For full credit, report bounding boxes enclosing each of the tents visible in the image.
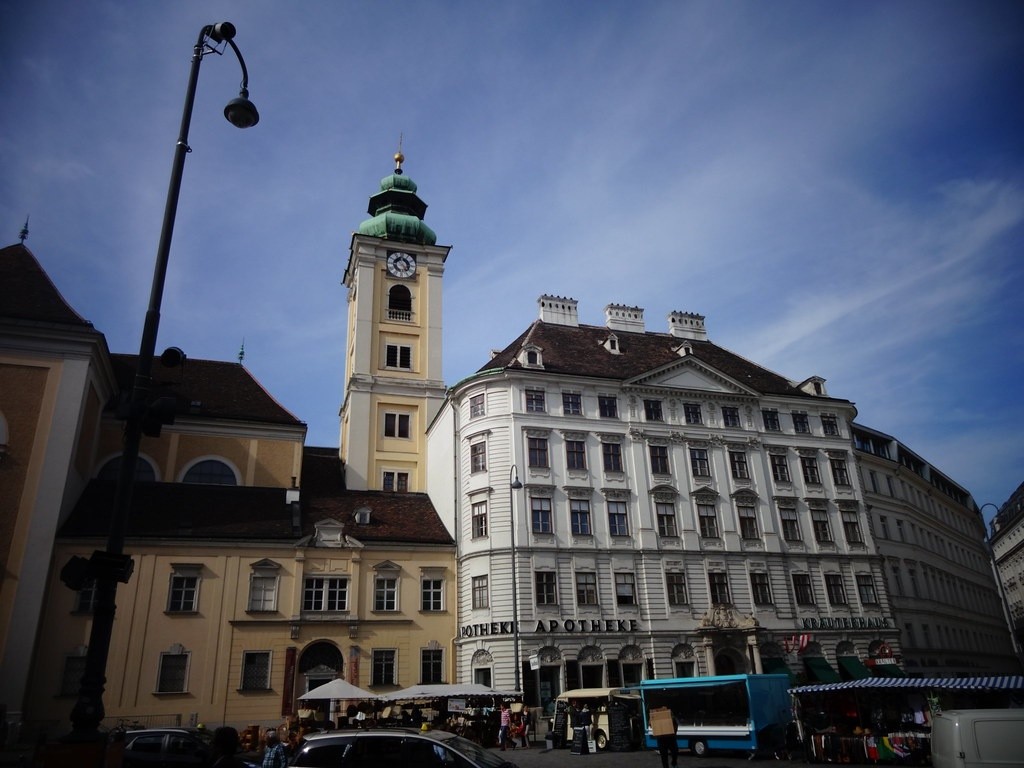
[377,683,524,701]
[788,676,1024,743]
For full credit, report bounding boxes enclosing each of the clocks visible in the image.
[386,252,416,278]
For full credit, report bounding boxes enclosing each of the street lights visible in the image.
[978,503,1024,665]
[509,464,523,702]
[66,19,260,768]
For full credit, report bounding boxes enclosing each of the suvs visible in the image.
[288,727,518,768]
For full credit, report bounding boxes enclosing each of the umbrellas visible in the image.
[297,679,376,722]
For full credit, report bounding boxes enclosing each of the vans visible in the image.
[548,688,641,748]
[930,707,1024,768]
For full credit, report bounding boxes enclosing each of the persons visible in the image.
[196,723,212,744]
[521,705,537,749]
[499,702,517,751]
[570,699,597,755]
[105,732,125,768]
[400,703,427,728]
[262,728,288,768]
[208,727,242,768]
[656,711,678,768]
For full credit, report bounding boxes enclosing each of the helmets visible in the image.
[197,723,205,728]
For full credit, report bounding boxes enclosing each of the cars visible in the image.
[117,730,257,768]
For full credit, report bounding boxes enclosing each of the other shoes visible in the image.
[512,742,517,750]
[499,747,505,751]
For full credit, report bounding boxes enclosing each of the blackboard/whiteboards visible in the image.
[570,730,585,754]
